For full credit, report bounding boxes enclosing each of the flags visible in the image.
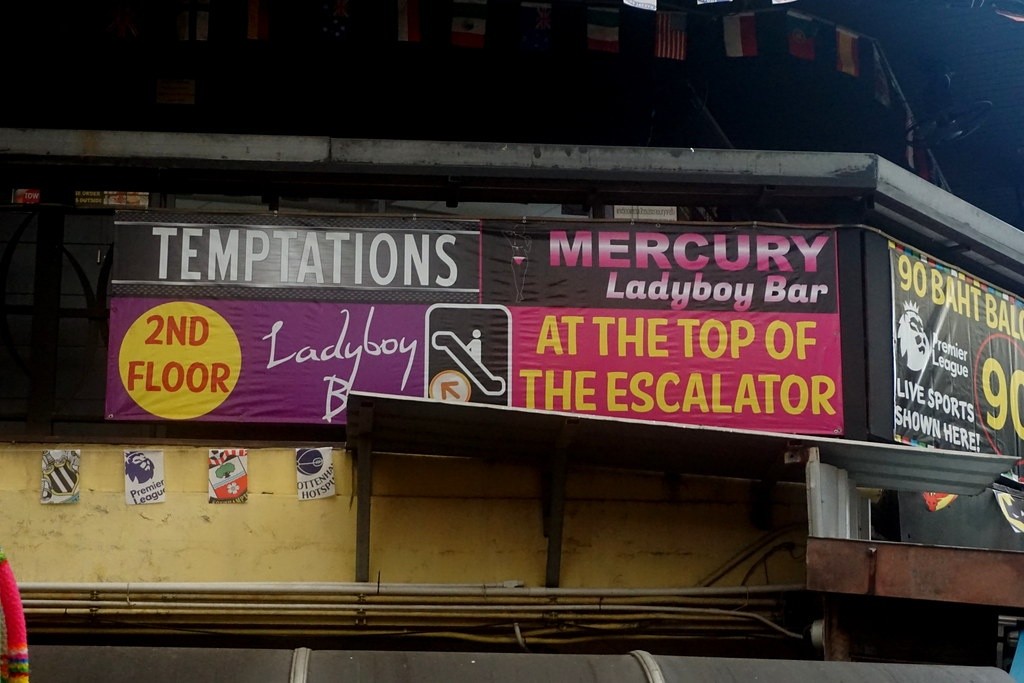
[722,12,758,58]
[872,45,931,181]
[449,0,490,50]
[321,0,353,39]
[785,11,816,60]
[834,27,861,77]
[656,10,688,60]
[519,0,554,53]
[111,0,278,43]
[586,6,621,53]
[397,0,428,45]
